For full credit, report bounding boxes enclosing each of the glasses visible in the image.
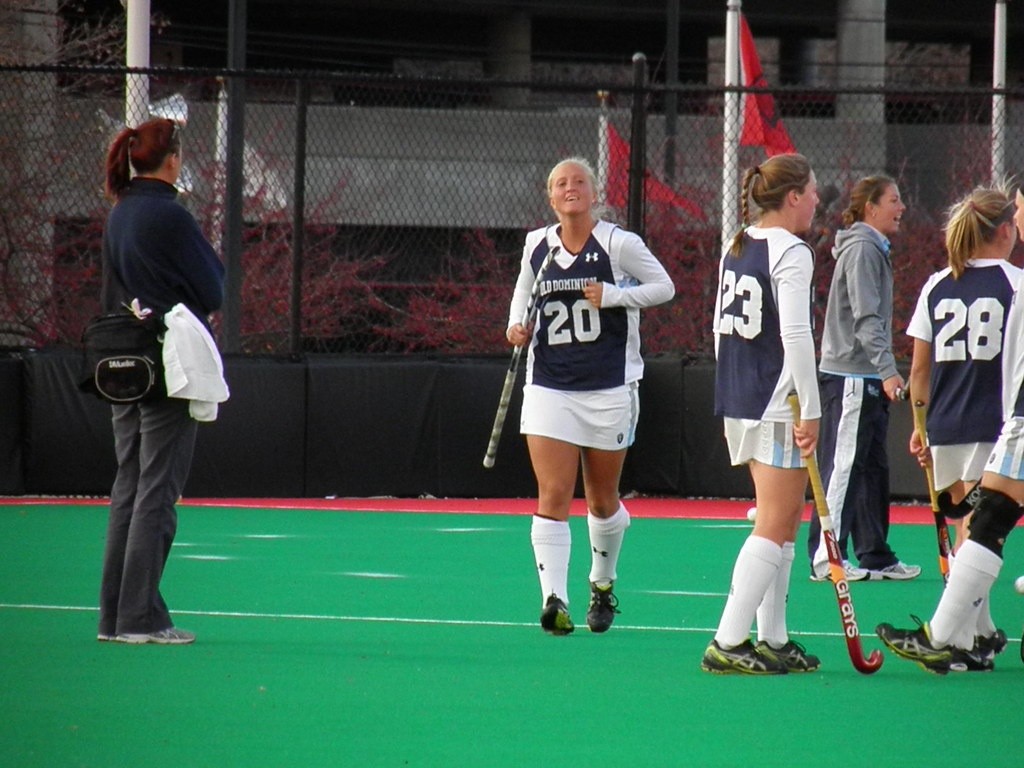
[167,118,180,144]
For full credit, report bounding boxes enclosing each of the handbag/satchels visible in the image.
[84,308,186,404]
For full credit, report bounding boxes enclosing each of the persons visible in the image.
[701,151,823,674]
[505,155,676,634]
[905,174,1024,672]
[874,178,1024,677]
[806,171,922,582]
[96,116,226,644]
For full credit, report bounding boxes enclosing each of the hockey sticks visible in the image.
[936,477,983,516]
[480,244,561,470]
[914,399,950,589]
[788,388,885,675]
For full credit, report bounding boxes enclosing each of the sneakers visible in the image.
[587,579,621,633]
[754,639,821,672]
[97,632,116,640]
[699,638,788,676]
[875,614,956,675]
[868,560,922,581]
[541,593,574,636]
[972,628,1007,657]
[810,560,871,582]
[949,644,993,672]
[117,626,195,644]
